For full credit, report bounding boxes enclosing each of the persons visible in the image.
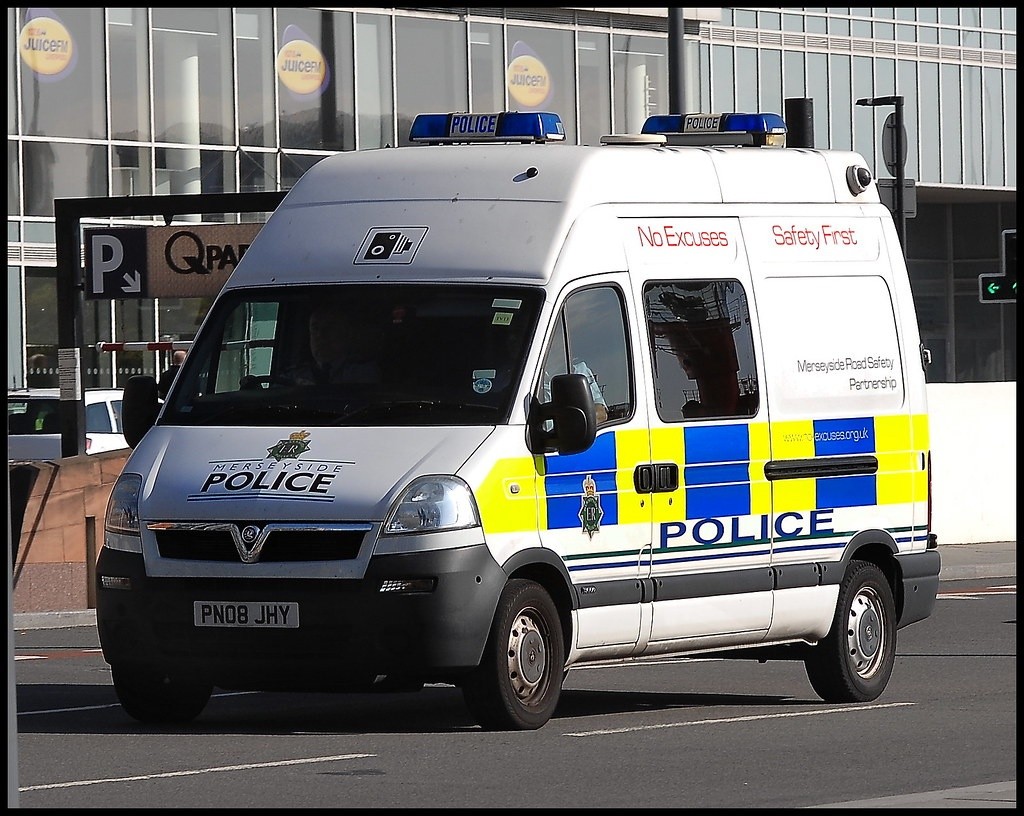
[543,334,610,427]
[158,350,187,412]
[239,300,381,390]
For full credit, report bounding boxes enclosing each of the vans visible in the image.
[93,110,941,732]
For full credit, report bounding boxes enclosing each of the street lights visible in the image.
[854,94,906,263]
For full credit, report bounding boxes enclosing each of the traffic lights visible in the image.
[978,231,1016,302]
